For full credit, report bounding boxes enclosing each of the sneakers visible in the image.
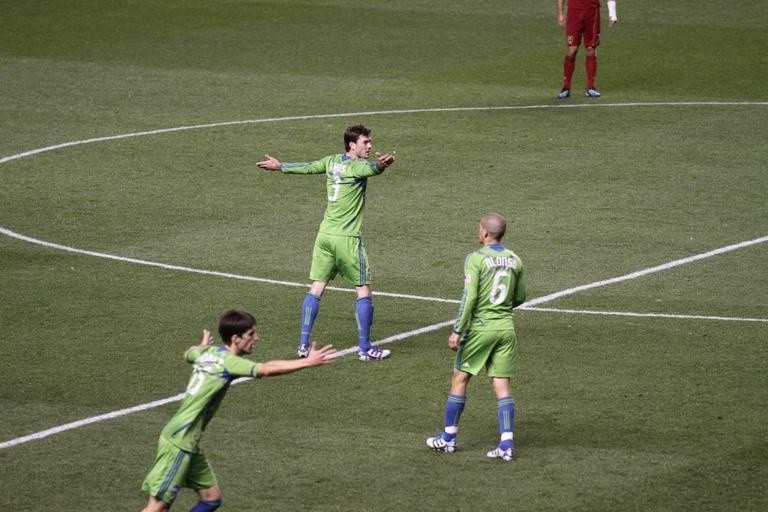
[426,436,458,454]
[356,344,392,361]
[486,446,516,461]
[585,88,601,97]
[296,343,323,358]
[557,88,570,99]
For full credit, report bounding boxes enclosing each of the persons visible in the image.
[139,306,337,512]
[253,123,396,363]
[553,0,618,100]
[424,211,526,461]
